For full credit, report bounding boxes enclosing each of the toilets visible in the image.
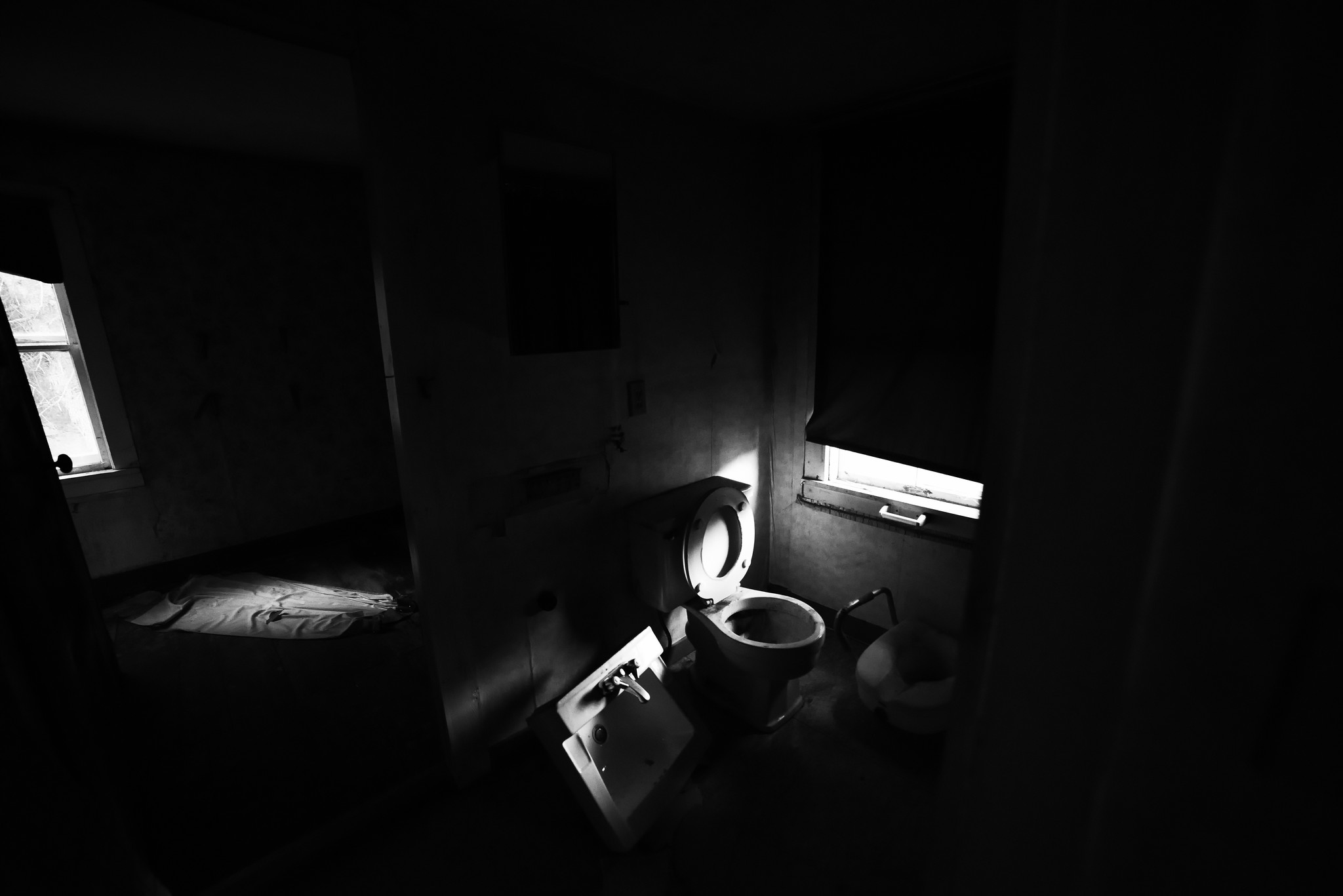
[625,476,826,736]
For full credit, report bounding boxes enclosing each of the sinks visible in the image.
[533,624,698,852]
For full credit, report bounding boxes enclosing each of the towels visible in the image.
[123,570,399,641]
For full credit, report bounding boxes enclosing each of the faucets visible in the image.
[599,659,652,705]
